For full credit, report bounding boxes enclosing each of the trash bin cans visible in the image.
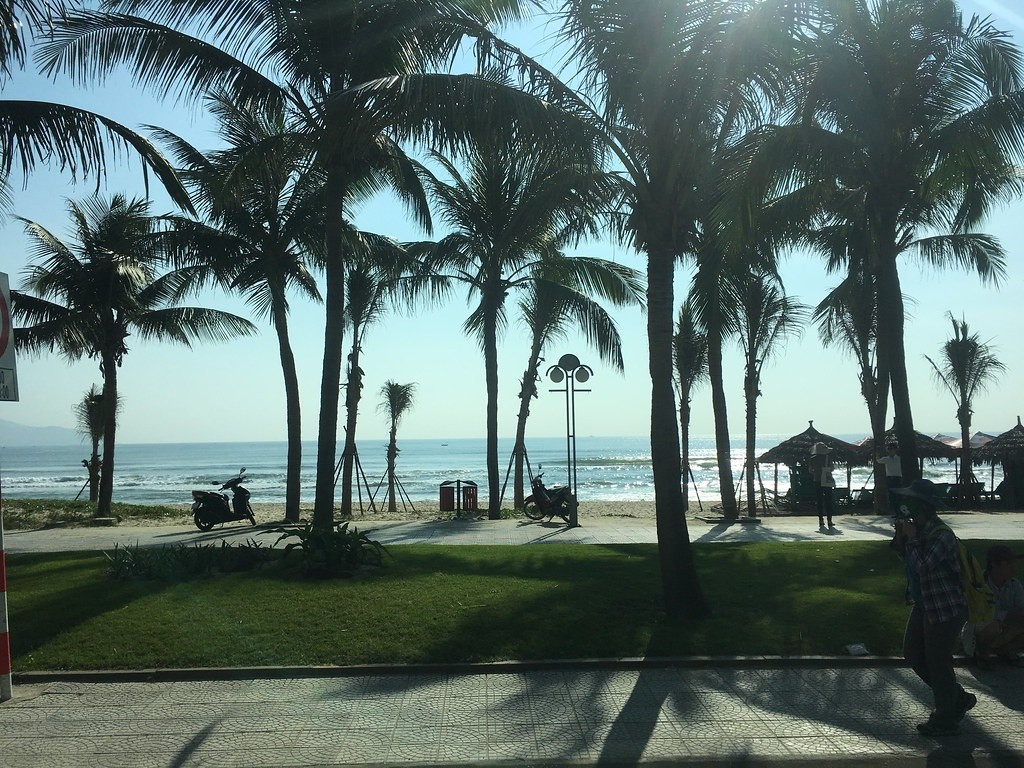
[440,480,478,511]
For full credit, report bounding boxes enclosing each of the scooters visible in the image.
[189,467,256,532]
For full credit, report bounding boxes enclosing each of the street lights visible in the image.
[544,354,594,528]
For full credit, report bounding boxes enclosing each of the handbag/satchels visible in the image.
[821,455,835,487]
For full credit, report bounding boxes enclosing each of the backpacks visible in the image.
[926,525,996,625]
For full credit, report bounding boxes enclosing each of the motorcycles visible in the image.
[522,462,579,523]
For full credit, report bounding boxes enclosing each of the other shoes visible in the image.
[1003,647,1024,667]
[828,522,835,526]
[917,719,960,736]
[819,522,825,525]
[929,684,976,722]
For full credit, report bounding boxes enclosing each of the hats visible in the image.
[889,479,935,504]
[985,544,1024,561]
[811,442,833,455]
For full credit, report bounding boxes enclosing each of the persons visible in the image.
[807,441,836,526]
[875,443,903,518]
[961,543,1024,668]
[889,478,977,735]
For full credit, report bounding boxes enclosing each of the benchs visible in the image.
[933,481,985,505]
[836,488,850,504]
